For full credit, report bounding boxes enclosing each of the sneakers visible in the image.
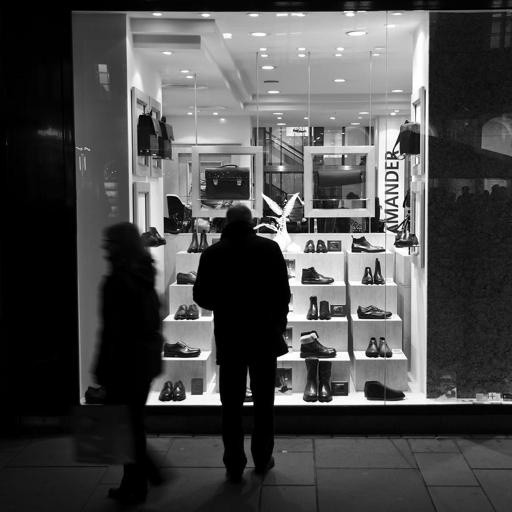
[225,455,274,483]
[108,486,147,503]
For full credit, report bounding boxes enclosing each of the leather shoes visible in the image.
[366,337,392,358]
[351,236,385,252]
[304,240,326,253]
[177,273,197,285]
[159,381,185,401]
[141,227,166,247]
[357,305,391,319]
[174,304,199,320]
[364,380,404,399]
[163,340,200,357]
[302,267,333,285]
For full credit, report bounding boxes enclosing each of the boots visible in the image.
[306,296,331,320]
[300,331,336,402]
[188,229,208,253]
[363,258,385,284]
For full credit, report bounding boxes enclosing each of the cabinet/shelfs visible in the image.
[144,233,408,406]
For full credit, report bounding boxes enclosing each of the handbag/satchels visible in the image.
[394,216,418,248]
[138,109,174,160]
[264,183,287,216]
[205,164,250,199]
[317,170,362,199]
[398,120,420,155]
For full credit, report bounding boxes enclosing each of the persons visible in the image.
[191,205,293,483]
[89,220,178,505]
[433,183,512,213]
